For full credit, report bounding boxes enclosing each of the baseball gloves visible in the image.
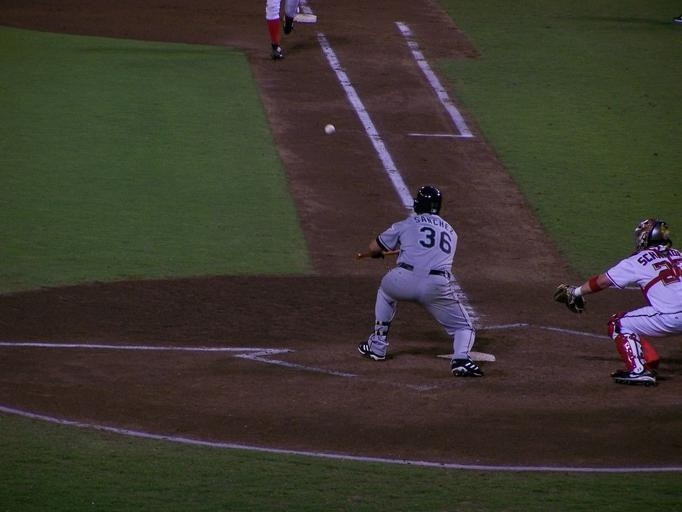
[553,283,587,313]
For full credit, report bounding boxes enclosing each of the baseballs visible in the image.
[325,124,335,134]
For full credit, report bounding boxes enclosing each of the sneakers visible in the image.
[450,358,484,377]
[271,43,283,58]
[357,341,387,361]
[283,20,294,34]
[610,369,657,386]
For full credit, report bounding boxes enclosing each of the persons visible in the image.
[566,218,682,386]
[265,0,300,60]
[357,185,484,377]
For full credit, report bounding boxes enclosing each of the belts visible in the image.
[399,262,446,277]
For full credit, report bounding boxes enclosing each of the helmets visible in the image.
[413,186,441,213]
[634,220,669,249]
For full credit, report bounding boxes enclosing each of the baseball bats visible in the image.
[356,249,400,258]
